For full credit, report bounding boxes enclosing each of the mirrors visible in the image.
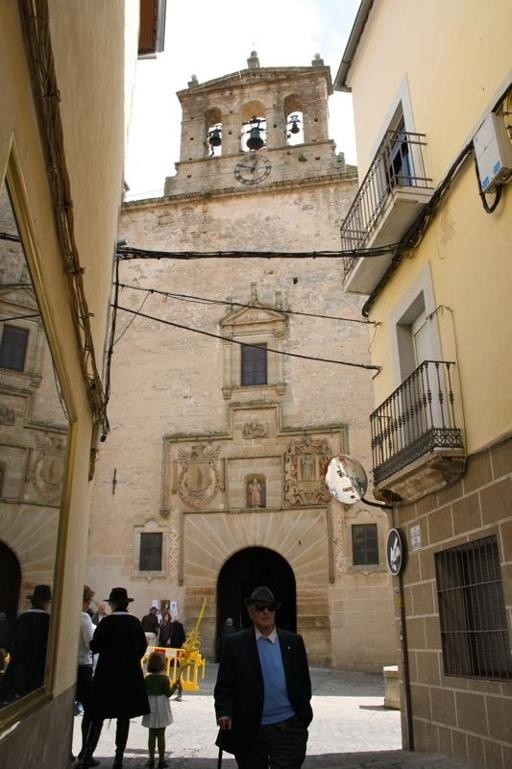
[324,455,368,505]
[0,135,81,734]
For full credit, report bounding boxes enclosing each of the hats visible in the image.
[103,587,135,603]
[26,584,53,600]
[244,587,278,606]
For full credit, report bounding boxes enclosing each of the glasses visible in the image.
[255,602,275,613]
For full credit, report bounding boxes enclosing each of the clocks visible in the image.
[233,153,271,185]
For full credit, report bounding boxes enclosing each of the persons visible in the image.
[0,583,190,768]
[301,452,316,482]
[248,476,265,509]
[210,584,316,769]
